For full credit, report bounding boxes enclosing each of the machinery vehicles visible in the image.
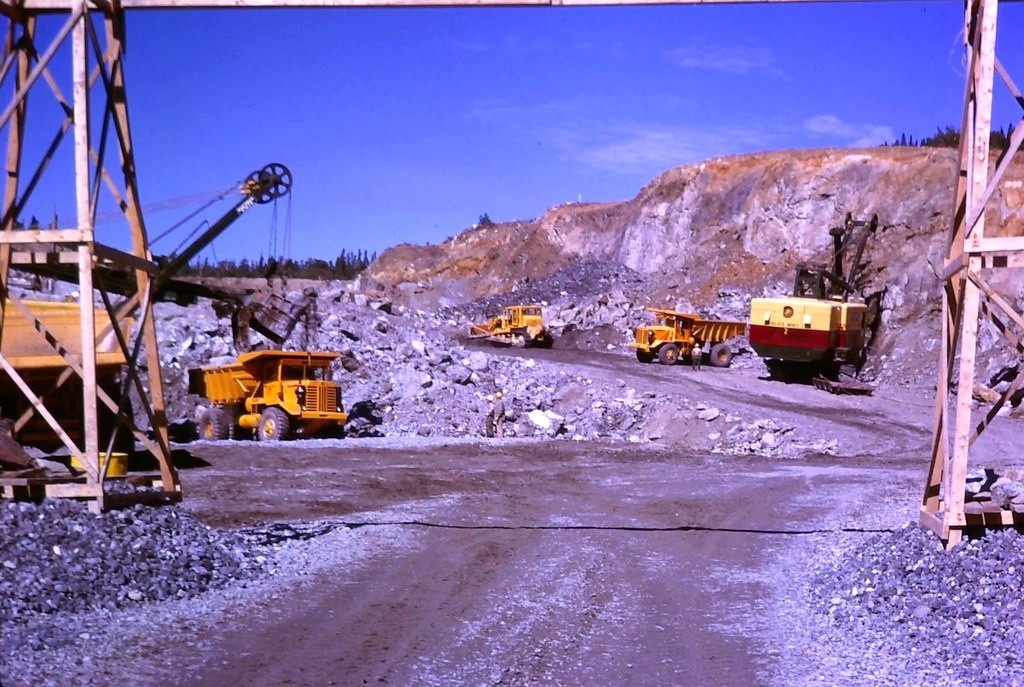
[466,305,547,349]
[628,306,748,368]
[748,211,886,385]
[184,346,349,445]
